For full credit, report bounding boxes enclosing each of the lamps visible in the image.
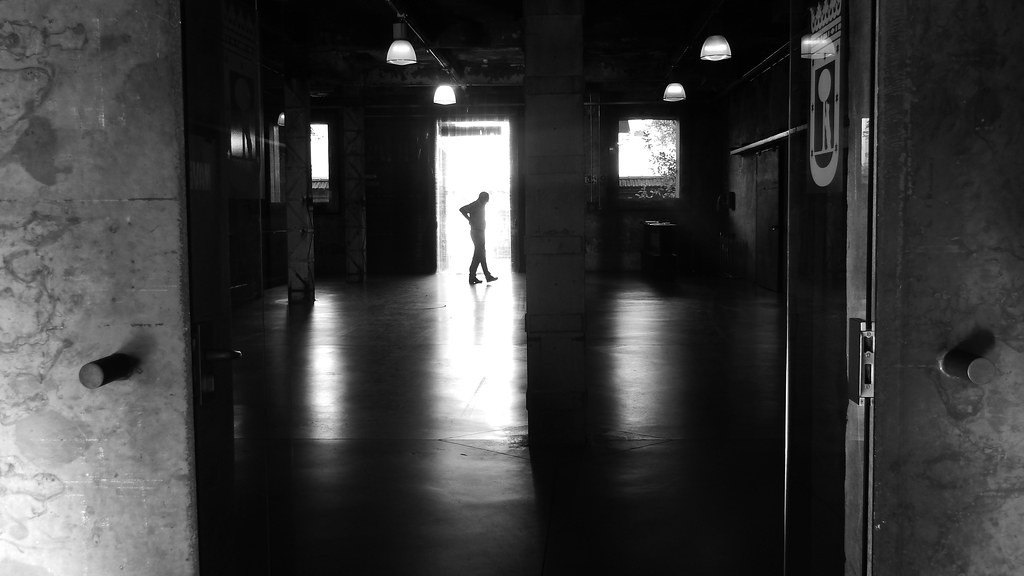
[433,67,457,105]
[700,8,732,61]
[386,13,417,65]
[661,63,686,103]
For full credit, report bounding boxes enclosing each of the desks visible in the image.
[641,224,677,273]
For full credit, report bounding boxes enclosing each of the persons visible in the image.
[459,192,498,285]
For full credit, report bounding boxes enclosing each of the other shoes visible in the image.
[469,277,482,283]
[486,275,498,282]
